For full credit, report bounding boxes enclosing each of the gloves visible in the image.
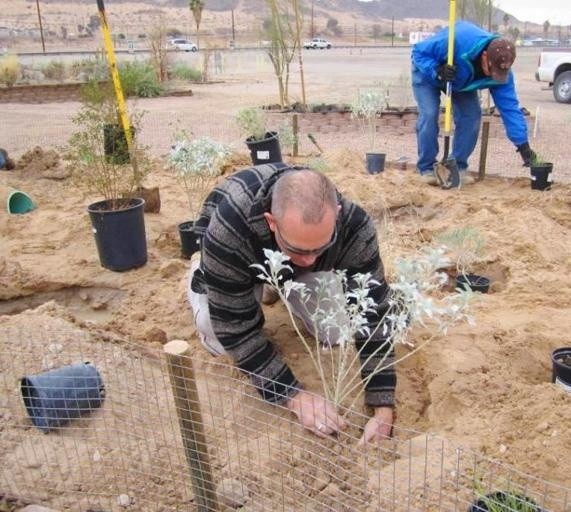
[436,64,457,82]
[516,142,537,167]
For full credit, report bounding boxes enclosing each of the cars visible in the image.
[166,39,197,52]
[536,51,571,103]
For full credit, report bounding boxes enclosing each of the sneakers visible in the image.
[423,169,475,186]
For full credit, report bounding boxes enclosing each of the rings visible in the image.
[318,424,324,430]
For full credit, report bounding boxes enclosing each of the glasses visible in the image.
[277,225,338,256]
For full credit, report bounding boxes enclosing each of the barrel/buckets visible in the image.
[0,148,15,171]
[455,274,492,294]
[366,152,386,174]
[245,133,281,166]
[89,197,147,270]
[527,162,554,191]
[178,221,199,257]
[103,123,135,166]
[7,191,34,215]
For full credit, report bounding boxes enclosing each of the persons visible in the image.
[411,20,537,187]
[182,161,398,446]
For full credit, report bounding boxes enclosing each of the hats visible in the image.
[486,37,516,82]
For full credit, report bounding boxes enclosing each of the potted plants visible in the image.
[469,480,543,511]
[68,63,151,273]
[435,226,491,293]
[351,88,392,175]
[528,151,554,192]
[161,133,230,258]
[233,102,283,166]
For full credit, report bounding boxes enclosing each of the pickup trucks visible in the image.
[304,38,331,50]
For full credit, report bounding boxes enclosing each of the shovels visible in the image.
[433,0,460,190]
[96,0,161,214]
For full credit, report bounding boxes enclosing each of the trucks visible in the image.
[410,32,434,45]
